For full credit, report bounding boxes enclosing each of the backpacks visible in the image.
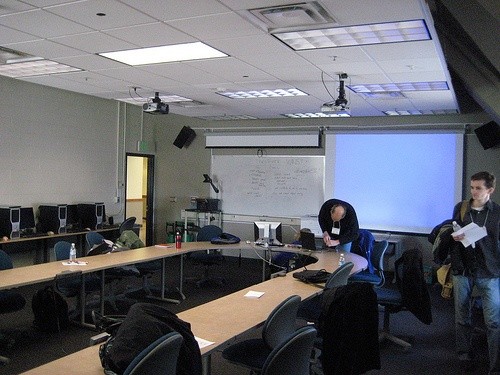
[32,285,69,330]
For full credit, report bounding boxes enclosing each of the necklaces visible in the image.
[470,209,489,226]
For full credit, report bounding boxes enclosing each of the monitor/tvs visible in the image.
[254,222,283,248]
[67,205,78,224]
[20,208,35,229]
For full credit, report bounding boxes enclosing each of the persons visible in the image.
[440,172,500,375]
[318,199,359,252]
[265,228,311,281]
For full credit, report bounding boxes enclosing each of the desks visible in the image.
[0,224,142,265]
[0,242,368,375]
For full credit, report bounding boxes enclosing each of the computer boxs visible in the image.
[39,205,67,234]
[78,204,103,230]
[0,208,20,240]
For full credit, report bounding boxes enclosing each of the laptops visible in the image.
[300,232,326,251]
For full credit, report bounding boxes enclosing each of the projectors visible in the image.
[143,102,169,115]
[321,105,351,115]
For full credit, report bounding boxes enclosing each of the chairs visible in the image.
[0,217,423,375]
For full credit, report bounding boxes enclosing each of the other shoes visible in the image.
[458,351,475,370]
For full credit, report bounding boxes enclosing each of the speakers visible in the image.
[173,126,197,150]
[473,121,500,150]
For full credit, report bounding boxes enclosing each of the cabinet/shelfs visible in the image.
[184,208,221,242]
[166,222,194,243]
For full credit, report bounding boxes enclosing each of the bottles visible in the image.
[338,253,346,267]
[452,221,461,232]
[70,243,76,263]
[175,232,181,249]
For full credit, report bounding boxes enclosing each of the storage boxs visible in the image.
[196,198,220,210]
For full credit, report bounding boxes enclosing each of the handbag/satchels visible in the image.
[293,269,332,284]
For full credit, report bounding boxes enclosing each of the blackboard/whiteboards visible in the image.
[210,155,326,217]
